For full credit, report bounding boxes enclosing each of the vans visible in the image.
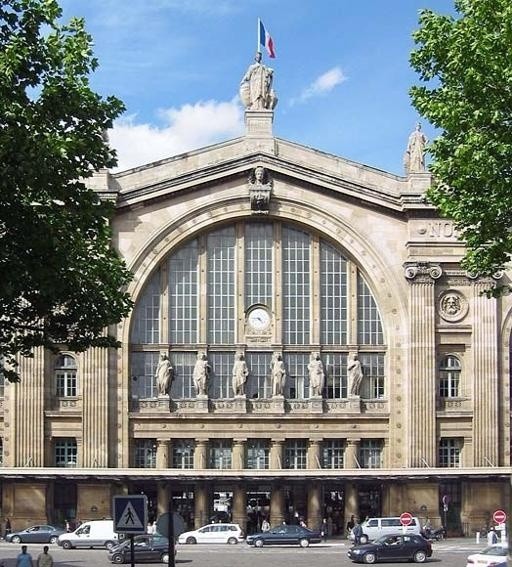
[349,517,422,544]
[57,521,122,550]
[178,523,245,545]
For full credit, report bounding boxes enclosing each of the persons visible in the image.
[305,352,325,396]
[485,525,499,546]
[347,354,364,396]
[77,520,83,533]
[153,351,173,396]
[442,295,460,314]
[65,519,74,532]
[270,354,286,396]
[252,165,265,185]
[146,512,434,546]
[1,517,12,539]
[36,545,54,566]
[193,351,209,399]
[243,52,278,109]
[405,122,430,171]
[16,544,35,566]
[230,351,249,396]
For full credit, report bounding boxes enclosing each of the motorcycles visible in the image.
[420,516,446,542]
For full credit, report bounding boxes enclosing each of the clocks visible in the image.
[244,302,273,335]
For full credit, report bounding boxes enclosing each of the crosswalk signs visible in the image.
[114,496,146,534]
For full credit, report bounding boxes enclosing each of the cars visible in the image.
[246,525,323,549]
[466,543,510,567]
[4,525,72,545]
[347,534,433,564]
[108,535,177,564]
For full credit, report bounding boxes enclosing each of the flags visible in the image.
[260,20,275,59]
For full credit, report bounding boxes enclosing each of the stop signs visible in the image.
[401,513,413,525]
[494,510,506,524]
